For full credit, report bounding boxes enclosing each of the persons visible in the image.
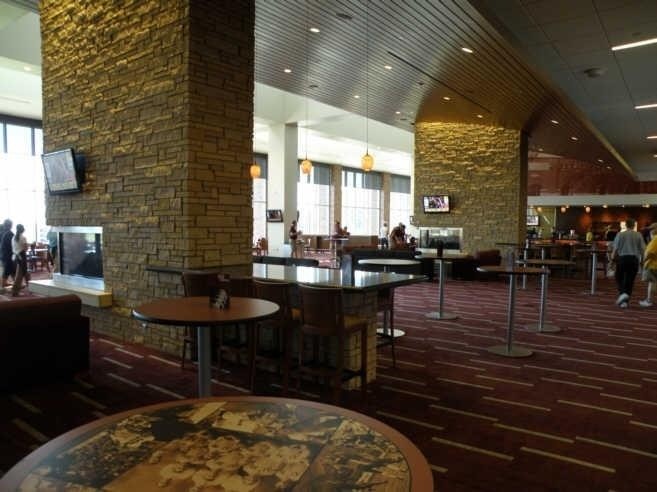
[334,221,343,238]
[608,217,646,308]
[405,237,416,260]
[0,219,26,293]
[9,223,27,297]
[635,228,656,309]
[341,226,350,238]
[389,224,406,249]
[604,223,617,265]
[45,229,57,266]
[287,220,301,259]
[640,219,651,249]
[379,223,388,250]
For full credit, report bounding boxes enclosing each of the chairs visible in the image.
[182,273,368,414]
[376,271,396,368]
[0,294,90,396]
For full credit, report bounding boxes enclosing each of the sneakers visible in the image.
[616,293,654,308]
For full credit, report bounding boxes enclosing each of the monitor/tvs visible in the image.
[41,149,81,195]
[423,194,451,214]
[526,215,540,226]
[266,209,283,222]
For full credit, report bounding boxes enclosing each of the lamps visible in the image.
[362,0,373,172]
[300,0,312,175]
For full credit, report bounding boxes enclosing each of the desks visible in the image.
[323,238,349,261]
[415,254,468,320]
[0,395,435,492]
[358,259,421,338]
[476,243,612,358]
[131,296,280,398]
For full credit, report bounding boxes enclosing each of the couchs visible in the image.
[351,248,502,281]
